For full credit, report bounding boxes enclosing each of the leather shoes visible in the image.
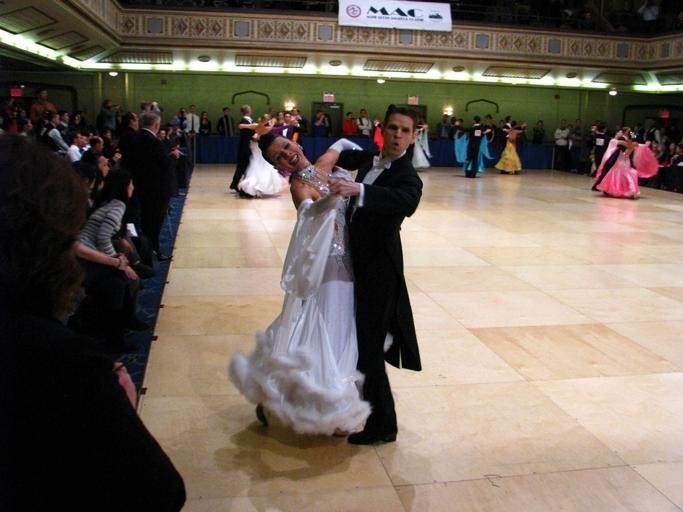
[348,427,398,446]
[230,184,239,192]
[158,253,172,262]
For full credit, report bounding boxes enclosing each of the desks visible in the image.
[197,133,557,169]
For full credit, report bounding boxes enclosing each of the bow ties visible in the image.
[373,155,392,171]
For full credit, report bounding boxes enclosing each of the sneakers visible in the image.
[110,339,140,354]
[133,263,155,280]
[126,316,150,333]
